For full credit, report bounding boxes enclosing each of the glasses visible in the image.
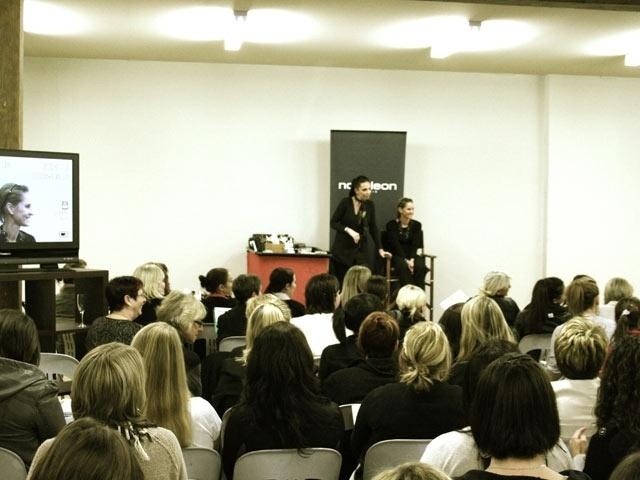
[194,320,204,327]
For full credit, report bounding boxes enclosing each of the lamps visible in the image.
[429,21,483,59]
[223,9,250,52]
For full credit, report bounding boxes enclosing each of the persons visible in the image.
[0,182,38,245]
[386,198,428,289]
[330,175,393,277]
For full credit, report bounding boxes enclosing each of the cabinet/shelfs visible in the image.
[0,264,110,362]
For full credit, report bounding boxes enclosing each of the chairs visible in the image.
[378,231,436,322]
[2,300,639,480]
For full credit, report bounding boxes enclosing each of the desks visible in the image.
[248,247,333,302]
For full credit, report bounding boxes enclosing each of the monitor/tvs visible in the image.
[0,148,81,273]
